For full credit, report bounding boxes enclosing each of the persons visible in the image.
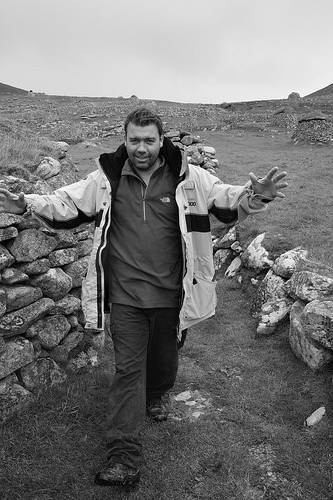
[0,108,288,487]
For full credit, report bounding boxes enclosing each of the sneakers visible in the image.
[96,463,140,486]
[148,394,168,421]
